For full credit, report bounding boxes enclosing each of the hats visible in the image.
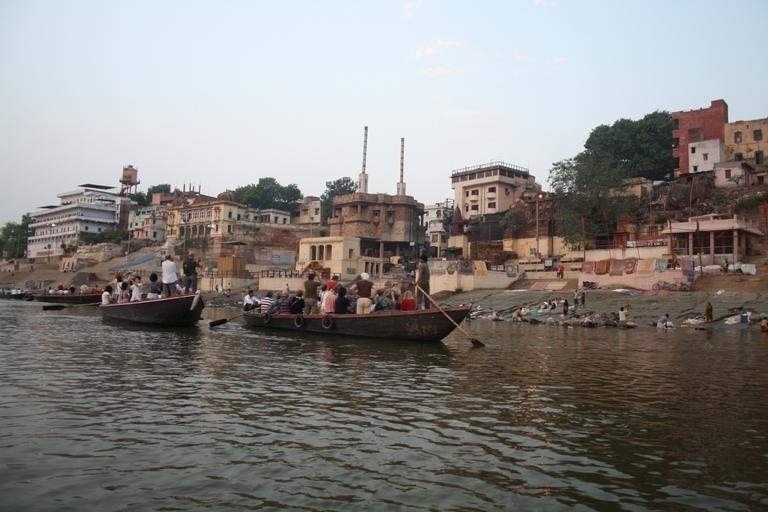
[361,272,369,280]
[418,255,427,261]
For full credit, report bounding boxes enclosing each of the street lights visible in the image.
[535,193,543,270]
[45,222,57,265]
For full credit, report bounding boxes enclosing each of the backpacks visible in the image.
[375,298,384,311]
[183,261,195,275]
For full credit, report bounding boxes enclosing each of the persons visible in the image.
[705,301,714,322]
[706,323,713,342]
[242,290,260,310]
[161,253,178,296]
[488,288,629,329]
[6,283,103,296]
[101,270,192,306]
[761,317,768,333]
[559,261,565,278]
[269,272,417,316]
[182,253,204,293]
[412,254,431,310]
[0,287,5,297]
[671,252,678,269]
[258,292,275,314]
[656,314,669,328]
